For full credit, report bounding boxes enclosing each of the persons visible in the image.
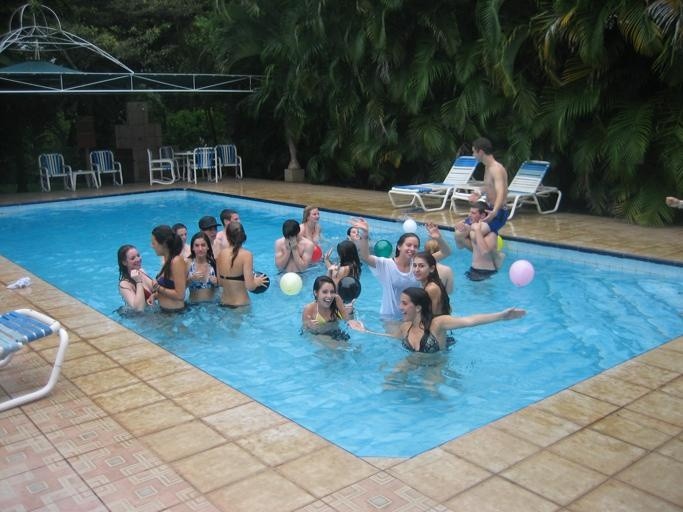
[116,209,269,314]
[324,240,362,291]
[347,287,527,391]
[666,196,683,210]
[345,216,451,317]
[299,206,322,245]
[301,276,355,348]
[347,227,361,241]
[454,201,498,280]
[454,136,509,268]
[275,220,314,273]
[411,251,452,316]
[424,239,454,295]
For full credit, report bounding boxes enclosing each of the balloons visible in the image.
[374,239,393,258]
[403,219,418,233]
[509,259,536,287]
[496,235,504,252]
[279,272,303,296]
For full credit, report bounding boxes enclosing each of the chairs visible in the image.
[39,153,74,192]
[388,156,562,220]
[0,309,68,411]
[147,144,243,186]
[89,151,123,187]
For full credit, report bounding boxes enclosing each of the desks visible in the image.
[72,171,99,192]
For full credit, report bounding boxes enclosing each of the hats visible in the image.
[198,215,222,230]
